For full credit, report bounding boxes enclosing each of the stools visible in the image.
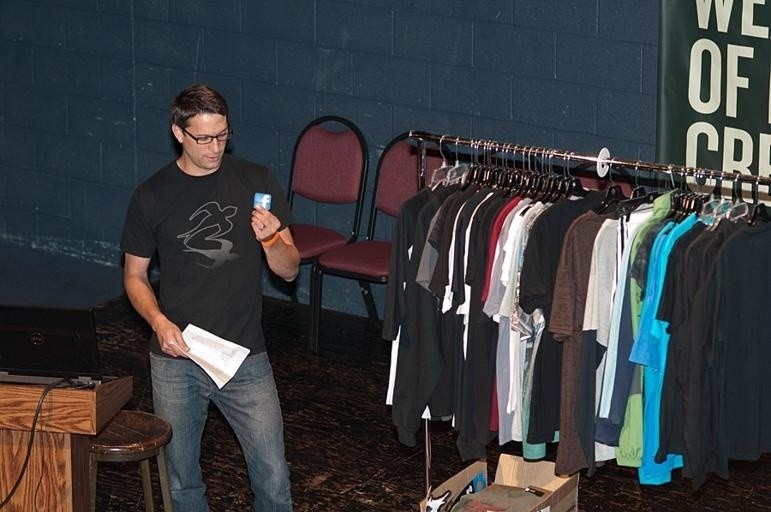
[87,410,173,512]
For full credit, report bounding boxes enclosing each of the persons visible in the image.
[121,83,301,512]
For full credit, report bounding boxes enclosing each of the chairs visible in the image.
[288,115,374,350]
[312,130,447,354]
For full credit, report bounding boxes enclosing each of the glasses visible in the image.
[179,126,234,146]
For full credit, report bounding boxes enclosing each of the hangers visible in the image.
[428,134,771,231]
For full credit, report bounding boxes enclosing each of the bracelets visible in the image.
[261,231,280,247]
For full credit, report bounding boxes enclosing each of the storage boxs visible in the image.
[419,453,580,512]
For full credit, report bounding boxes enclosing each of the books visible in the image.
[167,321,253,391]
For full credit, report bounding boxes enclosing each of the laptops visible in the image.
[0,303,118,387]
